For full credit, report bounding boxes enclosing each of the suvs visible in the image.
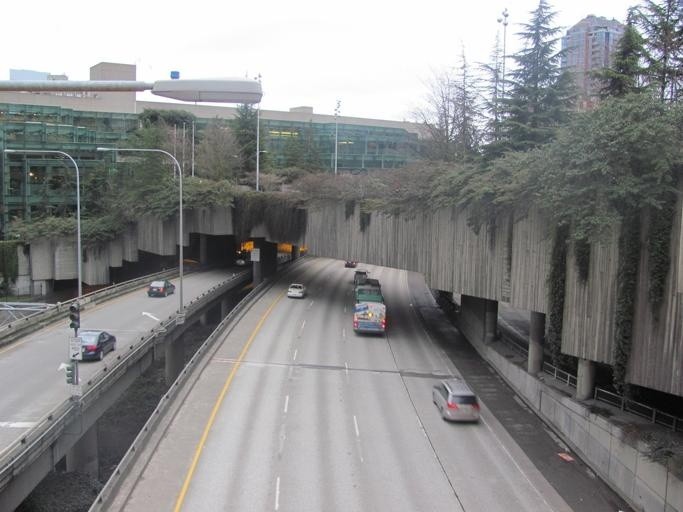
[432,378,481,426]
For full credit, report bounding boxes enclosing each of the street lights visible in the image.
[0,77,264,110]
[251,74,266,192]
[332,97,346,177]
[2,147,85,298]
[95,145,185,314]
[497,8,508,142]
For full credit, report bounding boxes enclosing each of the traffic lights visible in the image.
[69,302,78,330]
[65,365,72,383]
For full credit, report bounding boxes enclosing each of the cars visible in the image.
[344,260,358,268]
[76,329,116,363]
[145,280,175,297]
[287,283,306,298]
[352,268,386,335]
[231,249,251,267]
